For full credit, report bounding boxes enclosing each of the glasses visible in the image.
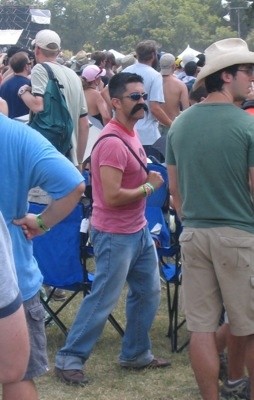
[233,68,254,75]
[119,93,148,100]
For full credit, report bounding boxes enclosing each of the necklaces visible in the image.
[88,86,95,89]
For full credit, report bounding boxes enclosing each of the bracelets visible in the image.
[35,214,50,232]
[141,183,154,197]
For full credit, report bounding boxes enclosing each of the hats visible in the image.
[31,29,61,51]
[160,53,176,75]
[192,38,254,91]
[82,65,106,83]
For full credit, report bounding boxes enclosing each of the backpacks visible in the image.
[29,63,74,155]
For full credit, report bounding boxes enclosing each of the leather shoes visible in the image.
[55,366,85,386]
[147,358,171,368]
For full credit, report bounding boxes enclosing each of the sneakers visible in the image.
[222,376,249,395]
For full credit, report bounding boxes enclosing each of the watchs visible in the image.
[18,88,29,98]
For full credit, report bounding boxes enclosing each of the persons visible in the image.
[153,53,190,159]
[188,86,208,107]
[79,65,111,172]
[164,37,254,400]
[17,29,90,301]
[0,112,86,400]
[0,51,32,123]
[53,72,171,385]
[0,210,31,385]
[0,74,9,117]
[215,301,249,400]
[0,49,254,116]
[120,39,173,164]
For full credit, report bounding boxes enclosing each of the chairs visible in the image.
[26,164,191,352]
